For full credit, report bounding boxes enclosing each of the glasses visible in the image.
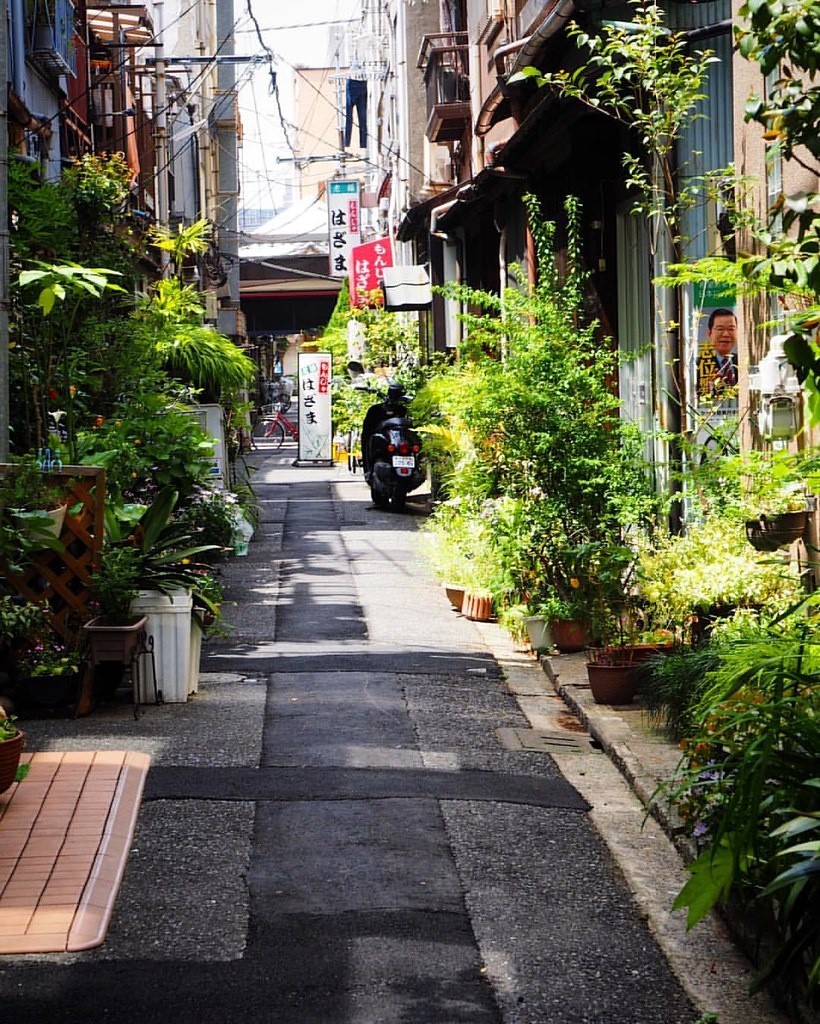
[712,325,736,334]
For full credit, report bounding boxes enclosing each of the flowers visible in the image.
[0,703,19,742]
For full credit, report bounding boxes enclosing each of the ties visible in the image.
[722,358,735,387]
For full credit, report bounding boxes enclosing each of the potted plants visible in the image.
[407,186,820,1023]
[81,547,151,719]
[1,147,263,704]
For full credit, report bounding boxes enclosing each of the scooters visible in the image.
[353,384,428,512]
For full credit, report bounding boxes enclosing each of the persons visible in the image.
[696,436,729,522]
[697,310,738,401]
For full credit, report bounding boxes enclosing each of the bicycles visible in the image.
[250,398,298,449]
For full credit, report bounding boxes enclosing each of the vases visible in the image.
[0,729,23,795]
